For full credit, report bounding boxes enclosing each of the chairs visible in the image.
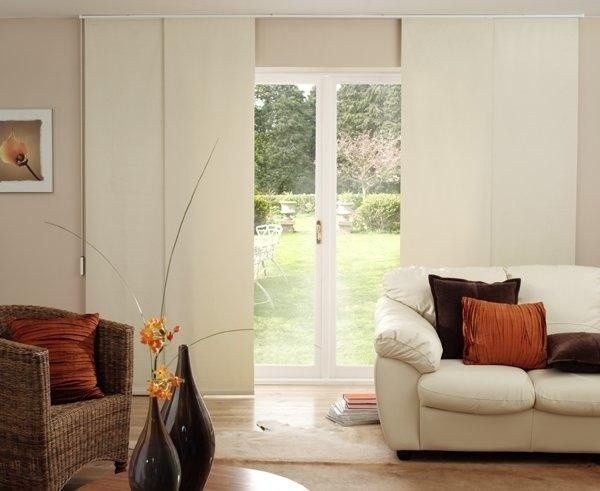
[0,301,137,490]
[254,223,289,310]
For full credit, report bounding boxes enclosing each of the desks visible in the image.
[75,461,312,490]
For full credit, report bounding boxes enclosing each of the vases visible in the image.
[160,343,218,491]
[124,393,187,491]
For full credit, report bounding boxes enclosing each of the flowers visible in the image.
[141,313,192,402]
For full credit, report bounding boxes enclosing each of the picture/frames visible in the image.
[0,106,57,192]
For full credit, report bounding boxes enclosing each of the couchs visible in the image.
[373,265,600,465]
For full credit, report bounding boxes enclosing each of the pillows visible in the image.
[6,312,105,403]
[428,273,522,363]
[545,331,600,375]
[458,294,549,368]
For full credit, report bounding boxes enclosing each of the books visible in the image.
[324,393,380,427]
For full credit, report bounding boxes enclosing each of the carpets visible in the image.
[129,419,598,490]
